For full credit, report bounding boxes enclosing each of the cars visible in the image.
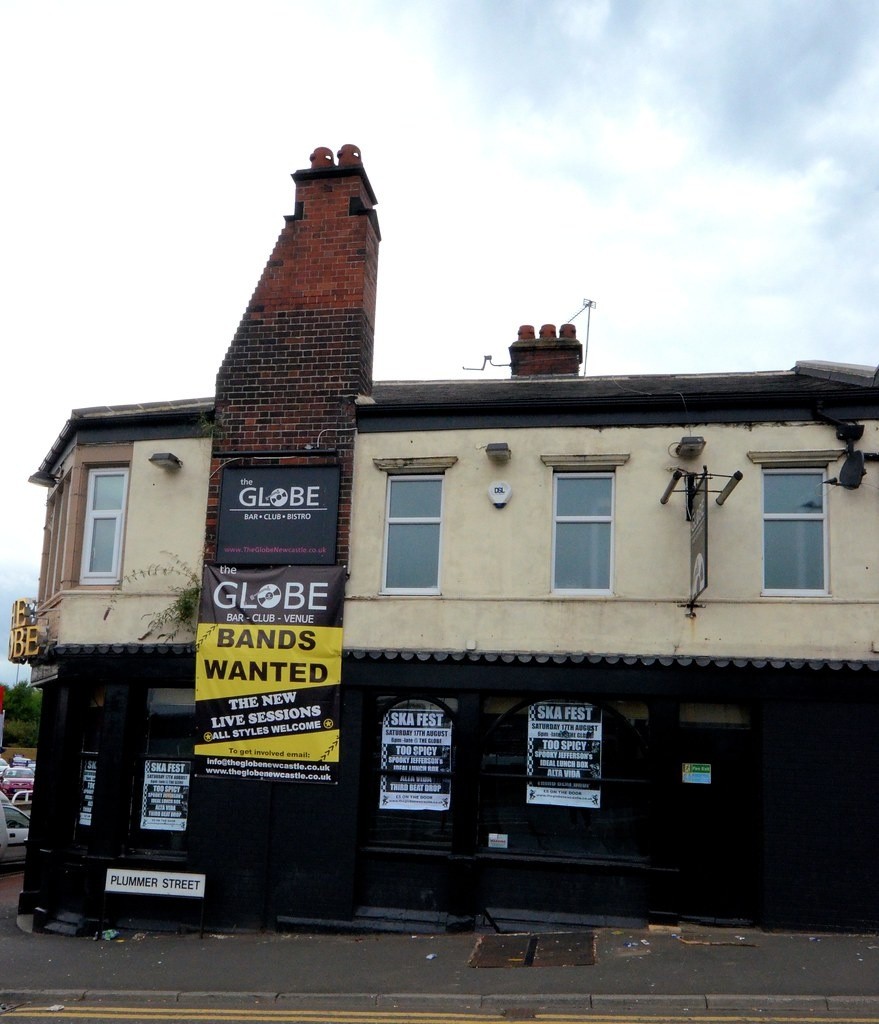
[0,806,30,865]
[0,758,10,779]
[10,755,37,773]
[0,767,35,800]
